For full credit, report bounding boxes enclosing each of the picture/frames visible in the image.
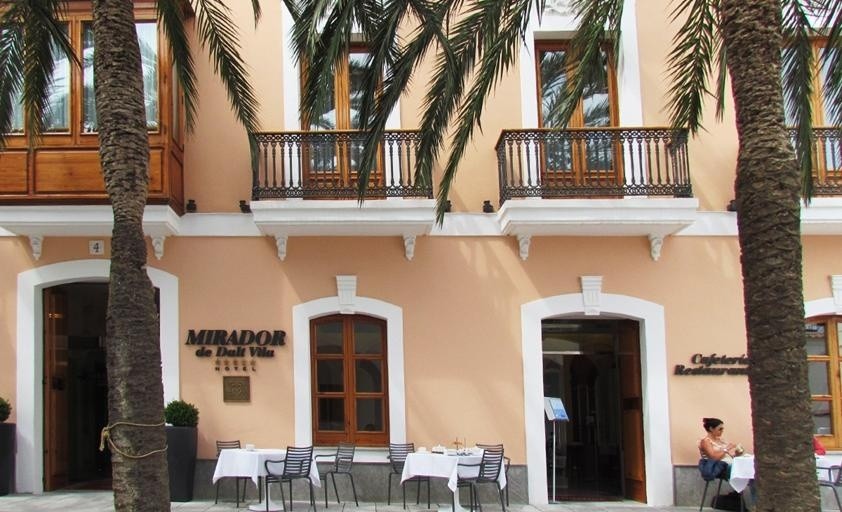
[223,376,250,401]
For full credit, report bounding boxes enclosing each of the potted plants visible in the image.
[164,400,199,502]
[0,398,16,495]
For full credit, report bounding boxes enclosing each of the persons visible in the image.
[813,437,825,456]
[698,417,736,480]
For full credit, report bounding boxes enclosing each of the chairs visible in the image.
[265,446,316,511]
[698,446,744,512]
[387,443,430,509]
[451,448,505,512]
[314,445,358,508]
[816,465,841,512]
[473,444,510,510]
[215,441,261,508]
